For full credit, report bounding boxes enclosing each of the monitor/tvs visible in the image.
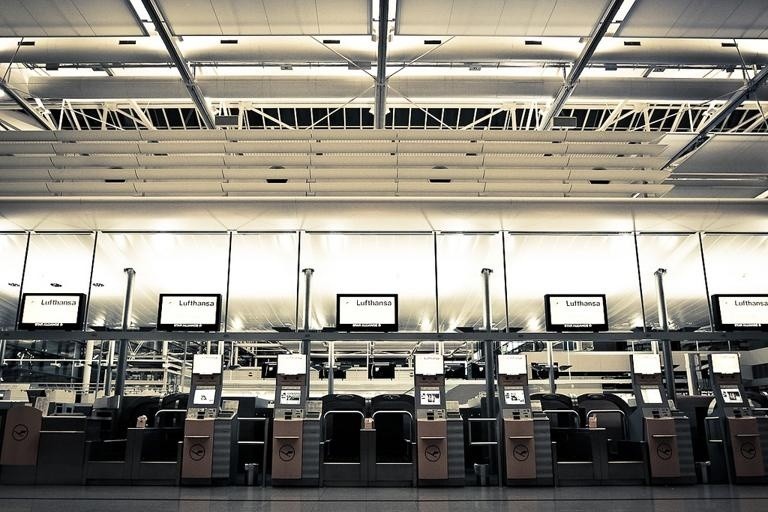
[262,364,277,378]
[319,365,346,378]
[720,388,744,404]
[640,388,663,404]
[444,362,465,378]
[701,360,710,379]
[472,362,486,378]
[95,394,121,417]
[27,389,46,407]
[504,390,526,404]
[420,390,441,406]
[532,362,558,379]
[193,389,216,405]
[280,390,301,405]
[368,362,394,378]
